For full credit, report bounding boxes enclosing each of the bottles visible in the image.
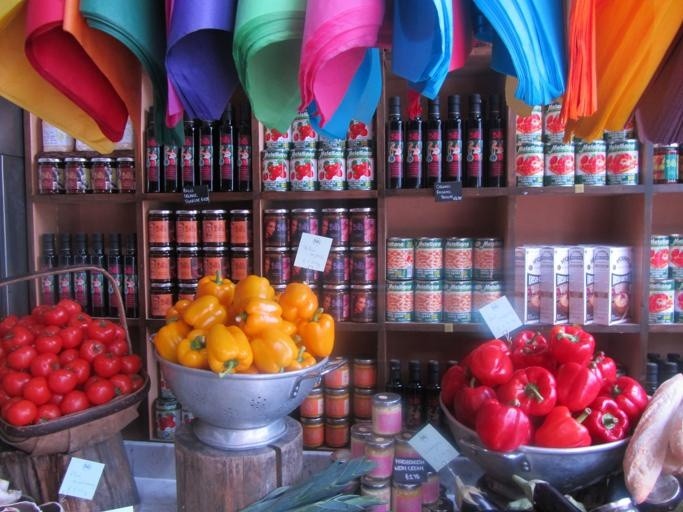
[386,358,460,428]
[145,102,254,194]
[38,231,139,320]
[385,89,509,189]
[643,351,682,397]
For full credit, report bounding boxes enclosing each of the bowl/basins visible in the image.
[142,334,346,449]
[439,394,634,502]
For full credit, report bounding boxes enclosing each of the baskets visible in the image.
[0,263,151,459]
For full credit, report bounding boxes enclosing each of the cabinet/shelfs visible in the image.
[22,49,683,453]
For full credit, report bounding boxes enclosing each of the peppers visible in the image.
[155,268,336,373]
[440,327,647,454]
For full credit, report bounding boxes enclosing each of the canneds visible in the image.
[641,475,683,511]
[653,142,680,184]
[516,103,640,187]
[152,366,195,441]
[37,156,138,194]
[261,110,375,191]
[148,209,254,317]
[263,207,376,324]
[302,358,440,511]
[386,237,502,325]
[648,233,683,325]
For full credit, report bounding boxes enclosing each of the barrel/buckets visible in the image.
[0,430,142,512]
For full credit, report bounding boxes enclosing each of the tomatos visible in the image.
[1,299,143,424]
